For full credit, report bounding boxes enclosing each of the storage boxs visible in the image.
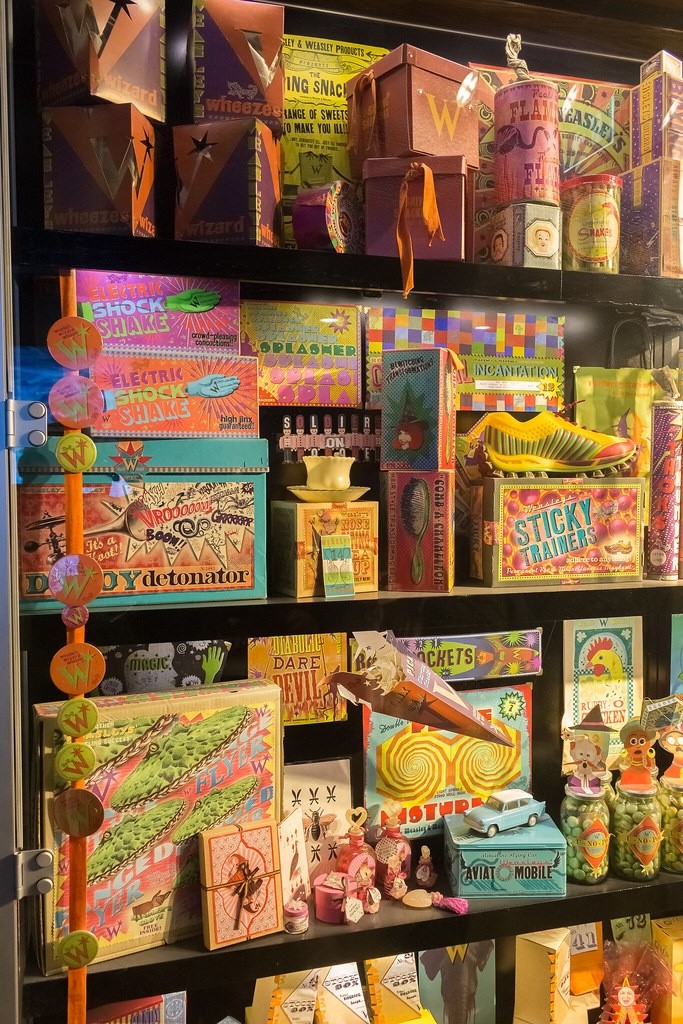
[78,911,683,1024]
[16,266,683,611]
[20,1,682,280]
[34,613,683,979]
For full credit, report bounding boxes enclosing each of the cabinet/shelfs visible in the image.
[0,0,683,1024]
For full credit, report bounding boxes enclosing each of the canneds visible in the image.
[559,769,683,886]
[493,79,622,275]
